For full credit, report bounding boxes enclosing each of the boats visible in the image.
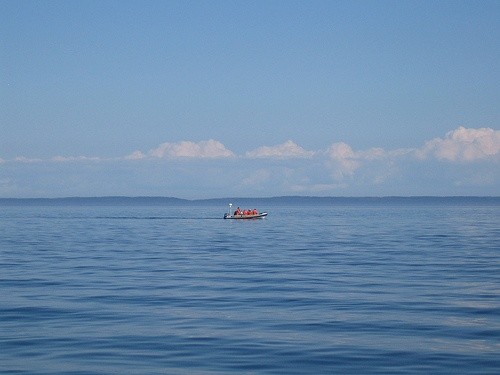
[225,203,268,219]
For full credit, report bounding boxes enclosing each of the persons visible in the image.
[253,208,259,214]
[235,206,254,215]
